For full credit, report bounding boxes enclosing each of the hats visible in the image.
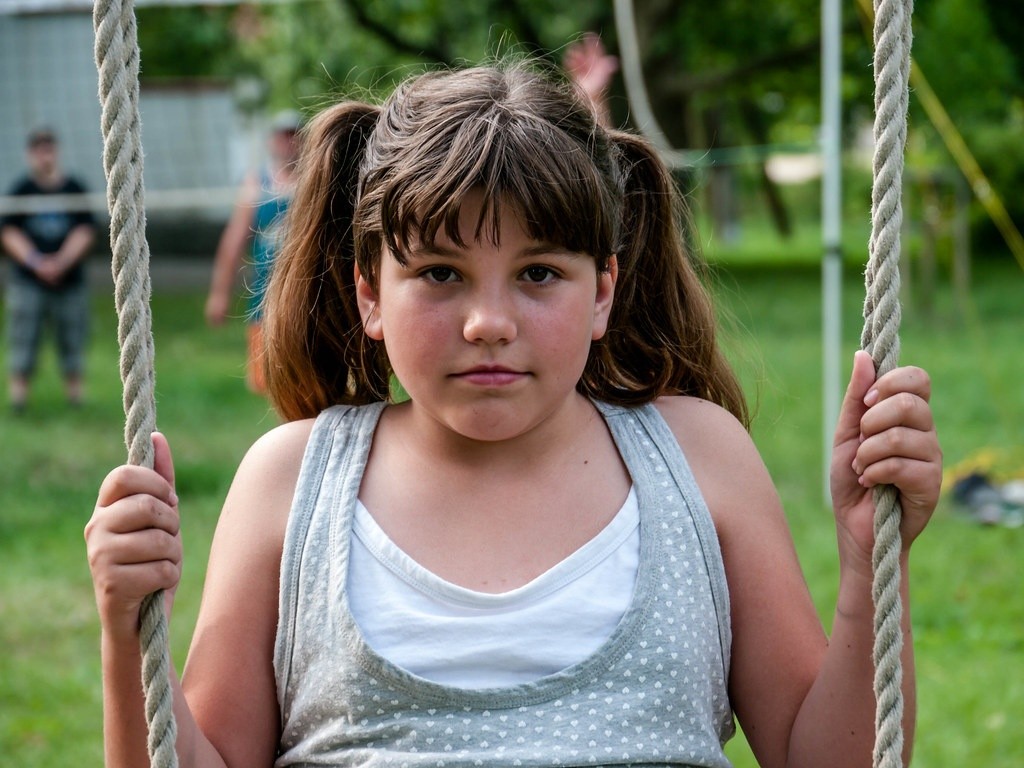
[272,108,302,133]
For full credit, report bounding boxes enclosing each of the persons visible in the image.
[84,58,942,768]
[0,125,102,410]
[202,110,306,394]
[565,32,621,132]
